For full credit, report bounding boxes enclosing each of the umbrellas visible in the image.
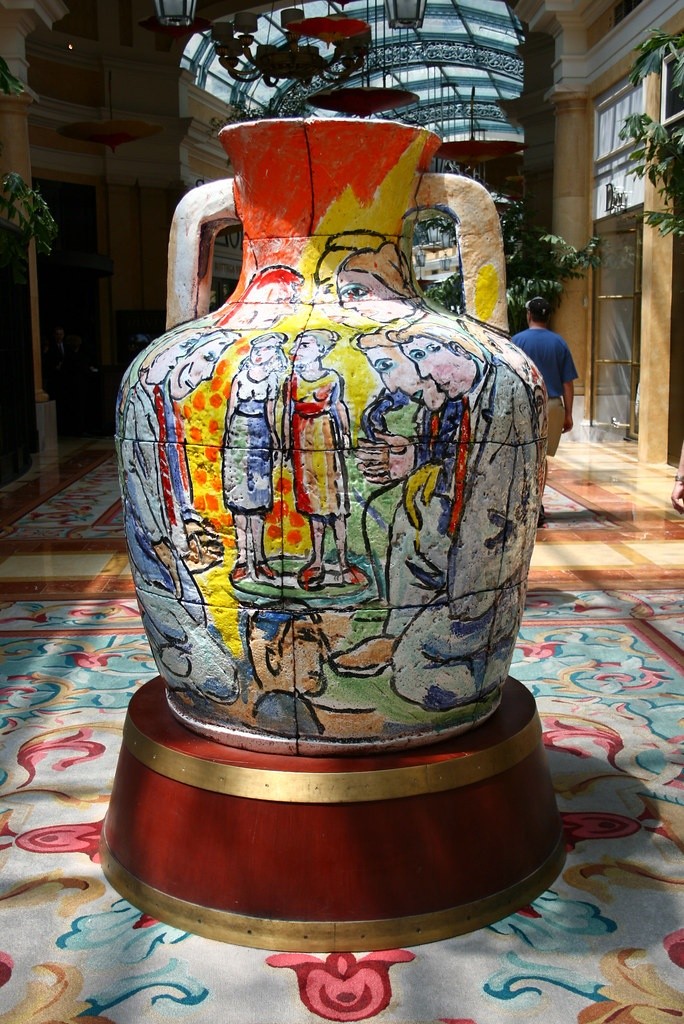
[305,9,416,116]
[286,0,370,46]
[432,85,529,168]
[57,66,164,154]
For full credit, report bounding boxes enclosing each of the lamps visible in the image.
[209,0,373,89]
[154,0,197,26]
[382,0,429,29]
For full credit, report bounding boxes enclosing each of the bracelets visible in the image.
[675,476,684,481]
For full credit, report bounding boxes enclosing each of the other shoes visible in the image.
[538,504,546,528]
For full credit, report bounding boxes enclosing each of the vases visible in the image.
[110,116,555,759]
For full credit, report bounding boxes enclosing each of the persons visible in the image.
[670,435,684,514]
[41,326,81,370]
[510,297,580,527]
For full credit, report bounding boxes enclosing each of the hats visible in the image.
[524,296,551,320]
